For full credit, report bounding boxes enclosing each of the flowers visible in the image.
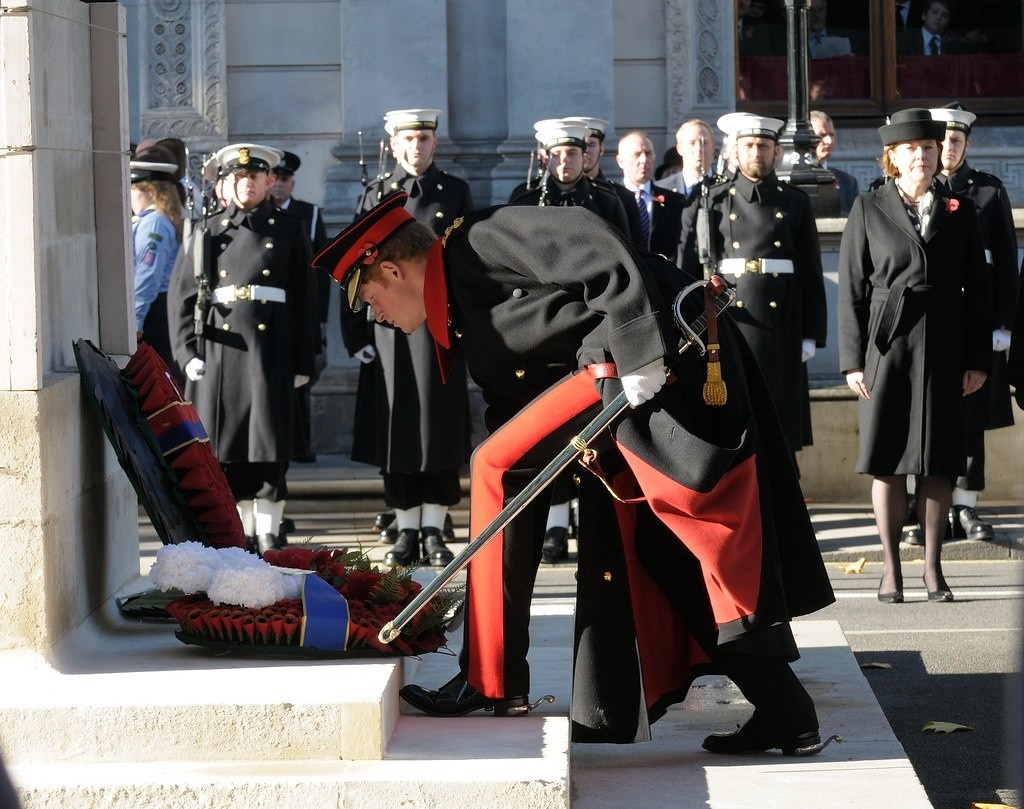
[120,340,466,656]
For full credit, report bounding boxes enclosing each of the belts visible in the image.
[714,259,794,275]
[209,285,288,303]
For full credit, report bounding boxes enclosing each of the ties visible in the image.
[814,34,823,43]
[895,5,904,31]
[633,189,651,249]
[930,38,939,54]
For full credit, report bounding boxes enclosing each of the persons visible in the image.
[737,0,998,101]
[507,111,859,562]
[837,100,1024,603]
[130,137,334,558]
[339,110,475,567]
[311,189,838,756]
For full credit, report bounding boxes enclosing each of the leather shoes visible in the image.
[242,516,295,556]
[399,672,528,718]
[701,712,822,757]
[878,574,905,603]
[904,493,994,545]
[923,572,953,601]
[540,535,568,561]
[374,508,455,567]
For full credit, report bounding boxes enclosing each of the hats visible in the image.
[383,109,442,135]
[717,112,784,141]
[130,146,179,184]
[878,109,947,145]
[533,116,609,154]
[201,143,301,175]
[655,146,682,179]
[926,101,977,134]
[307,188,417,313]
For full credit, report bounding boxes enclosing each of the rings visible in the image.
[979,384,982,387]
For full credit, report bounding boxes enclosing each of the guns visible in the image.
[375,136,390,202]
[694,131,717,280]
[183,145,220,235]
[194,154,213,359]
[527,141,556,207]
[357,128,371,187]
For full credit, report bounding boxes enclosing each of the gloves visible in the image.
[294,375,310,389]
[801,339,815,362]
[354,344,377,363]
[185,356,206,383]
[992,329,1011,352]
[618,357,667,407]
